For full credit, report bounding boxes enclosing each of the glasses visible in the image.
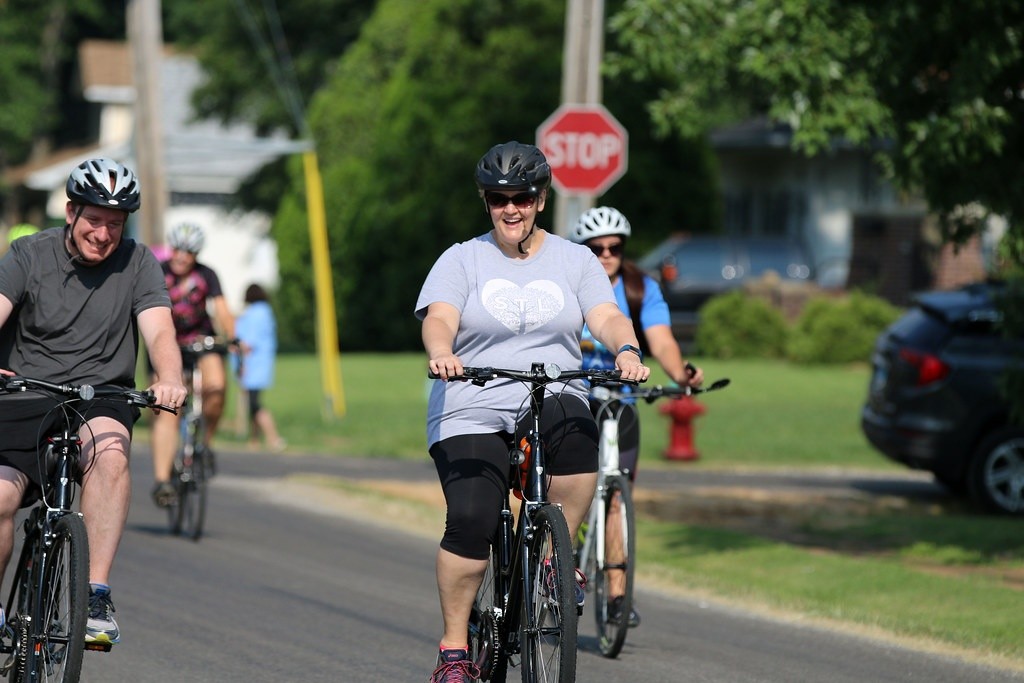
[582,241,628,259]
[484,191,544,210]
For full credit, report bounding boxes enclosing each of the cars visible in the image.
[642,231,851,343]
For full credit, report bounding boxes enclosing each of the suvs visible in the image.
[860,289,1024,520]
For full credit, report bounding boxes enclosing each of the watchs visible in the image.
[618,343,643,363]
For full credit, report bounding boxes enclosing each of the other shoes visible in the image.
[151,479,174,507]
[200,448,218,482]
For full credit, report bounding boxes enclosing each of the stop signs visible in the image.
[535,106,629,197]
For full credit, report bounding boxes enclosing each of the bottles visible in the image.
[513,437,531,500]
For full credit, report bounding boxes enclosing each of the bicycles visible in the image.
[0,372,190,683]
[167,336,240,540]
[536,361,730,659]
[427,363,648,683]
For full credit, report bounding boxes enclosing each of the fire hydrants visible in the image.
[657,394,709,459]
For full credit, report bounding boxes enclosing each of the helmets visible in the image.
[573,205,631,243]
[168,223,204,253]
[66,156,141,212]
[476,142,551,189]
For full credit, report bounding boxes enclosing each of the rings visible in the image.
[170,400,176,403]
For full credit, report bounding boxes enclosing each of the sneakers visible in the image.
[85,582,119,645]
[428,643,481,683]
[604,593,641,627]
[538,559,585,606]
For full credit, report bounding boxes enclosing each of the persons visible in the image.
[936,200,1008,289]
[227,283,287,453]
[148,226,243,508]
[412,140,651,683]
[566,202,702,625]
[0,158,187,676]
